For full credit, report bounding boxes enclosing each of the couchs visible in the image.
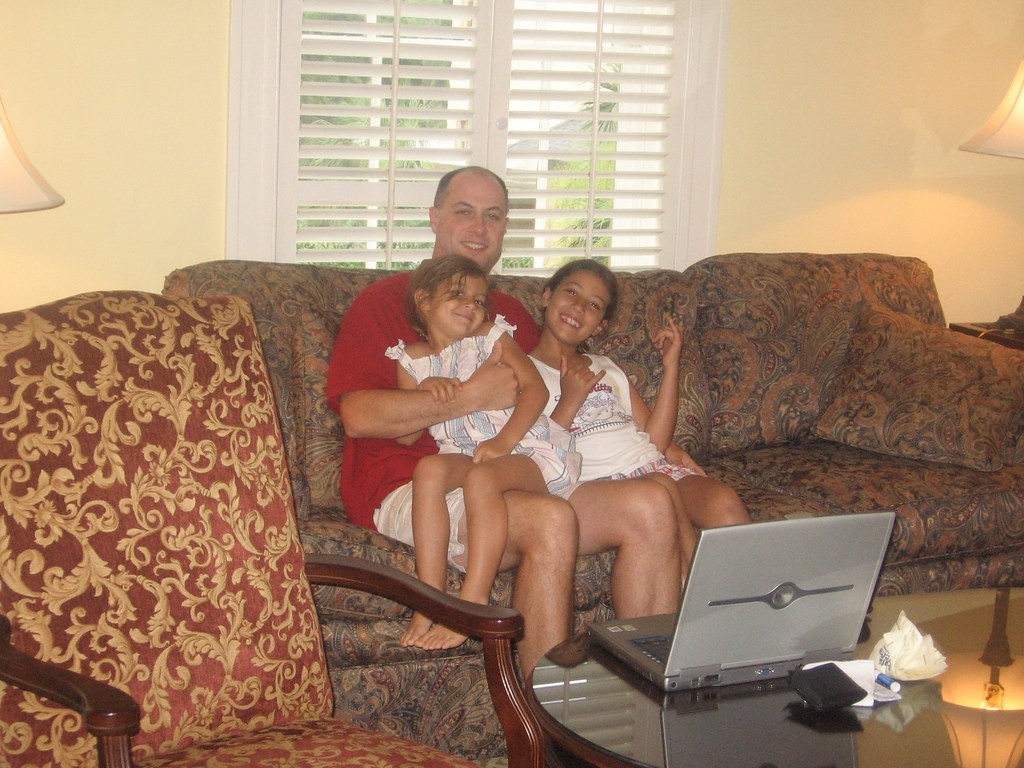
[163,252,1024,768]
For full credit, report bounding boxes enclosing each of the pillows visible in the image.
[810,293,1024,470]
[291,306,346,508]
[707,290,862,454]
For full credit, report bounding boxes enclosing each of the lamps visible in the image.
[935,585,1024,768]
[959,60,1024,333]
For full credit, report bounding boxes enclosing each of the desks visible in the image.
[949,323,1024,352]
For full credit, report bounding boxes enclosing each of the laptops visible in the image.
[587,509,897,692]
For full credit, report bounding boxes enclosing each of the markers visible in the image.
[875,667,902,694]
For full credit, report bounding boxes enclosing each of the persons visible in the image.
[384,256,583,651]
[523,258,754,595]
[326,167,683,683]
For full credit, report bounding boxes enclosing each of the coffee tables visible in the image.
[525,542,1024,768]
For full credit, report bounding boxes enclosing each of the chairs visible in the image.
[0,290,552,768]
